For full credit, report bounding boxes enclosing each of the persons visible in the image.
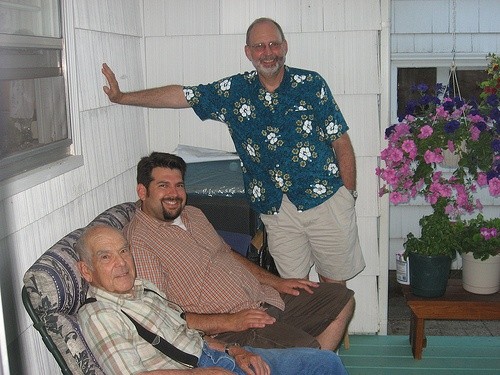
[102,18,366,287]
[120,151,357,350]
[75,224,348,375]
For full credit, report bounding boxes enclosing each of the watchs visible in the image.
[225,342,240,357]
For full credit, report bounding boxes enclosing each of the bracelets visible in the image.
[350,190,358,200]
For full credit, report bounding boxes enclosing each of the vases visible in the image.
[403,248,456,299]
[461,249,500,294]
[435,137,467,168]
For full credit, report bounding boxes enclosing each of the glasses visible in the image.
[249,41,285,50]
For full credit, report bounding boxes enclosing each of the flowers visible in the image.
[375,50,500,261]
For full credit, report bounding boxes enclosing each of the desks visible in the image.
[403,278,500,360]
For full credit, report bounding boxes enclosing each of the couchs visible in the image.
[20,201,147,375]
